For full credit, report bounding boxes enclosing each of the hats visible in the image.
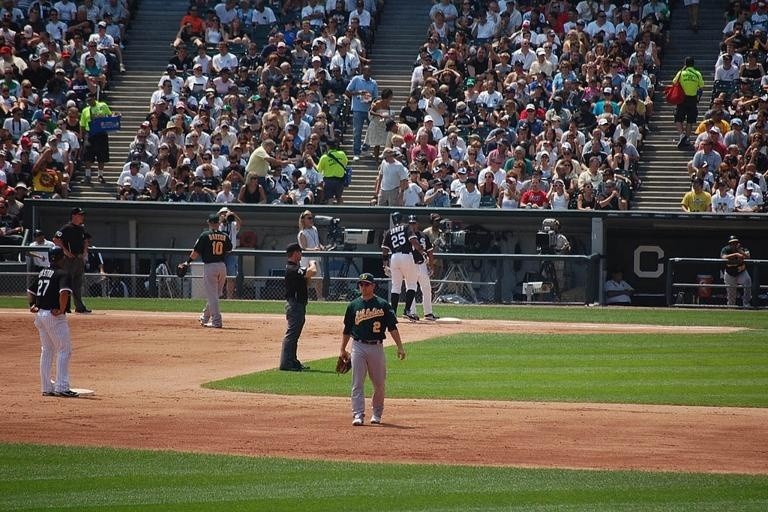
[0,7,113,190]
[727,235,739,243]
[380,7,644,192]
[285,242,304,251]
[70,206,86,216]
[205,213,219,225]
[131,18,354,166]
[687,2,768,193]
[356,272,375,285]
[216,206,233,215]
[430,212,444,223]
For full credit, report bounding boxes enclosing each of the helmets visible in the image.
[391,211,402,224]
[46,246,66,263]
[407,215,418,225]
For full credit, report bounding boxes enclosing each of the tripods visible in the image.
[335,257,361,291]
[432,260,478,304]
[539,259,560,302]
[158,264,179,299]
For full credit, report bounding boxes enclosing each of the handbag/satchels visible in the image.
[662,81,688,106]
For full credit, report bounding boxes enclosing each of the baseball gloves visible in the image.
[335,354,352,374]
[176,262,188,280]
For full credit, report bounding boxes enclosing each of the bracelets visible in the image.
[187,257,193,264]
[382,255,388,261]
[30,302,35,307]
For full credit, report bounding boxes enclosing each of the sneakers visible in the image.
[280,363,311,372]
[369,413,382,424]
[42,388,79,398]
[199,316,222,328]
[350,413,365,426]
[400,310,418,323]
[64,308,94,315]
[423,313,439,320]
[676,132,687,149]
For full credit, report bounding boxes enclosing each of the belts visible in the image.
[353,338,383,345]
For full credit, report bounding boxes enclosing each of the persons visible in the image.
[53,207,92,315]
[27,245,78,397]
[185,213,233,327]
[404,214,439,321]
[339,272,405,425]
[278,242,317,370]
[381,212,430,324]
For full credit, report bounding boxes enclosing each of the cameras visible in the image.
[224,215,234,224]
